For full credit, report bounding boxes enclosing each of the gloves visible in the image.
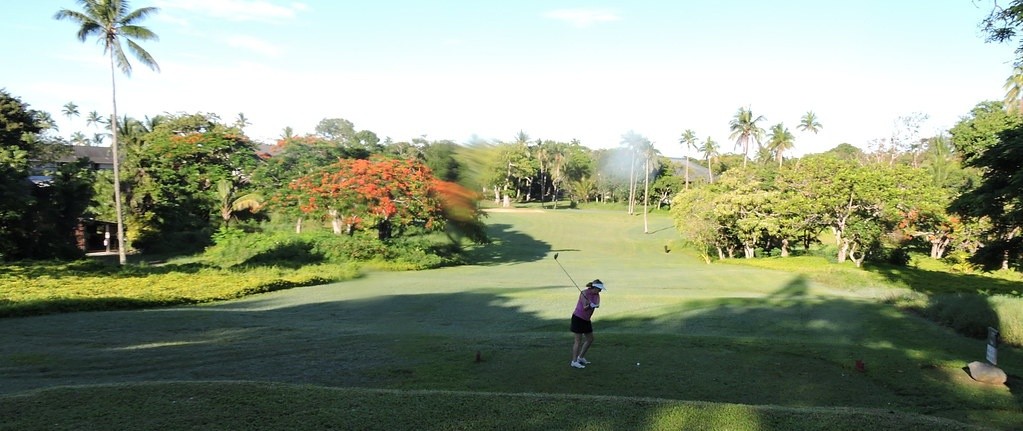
[590,303,596,308]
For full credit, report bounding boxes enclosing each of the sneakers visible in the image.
[571,361,585,368]
[577,356,591,364]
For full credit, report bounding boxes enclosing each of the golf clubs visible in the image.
[553,254,593,302]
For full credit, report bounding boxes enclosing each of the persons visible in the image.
[570,279,607,369]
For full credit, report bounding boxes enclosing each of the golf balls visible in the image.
[637,362,641,365]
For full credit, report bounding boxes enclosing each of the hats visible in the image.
[592,284,607,291]
